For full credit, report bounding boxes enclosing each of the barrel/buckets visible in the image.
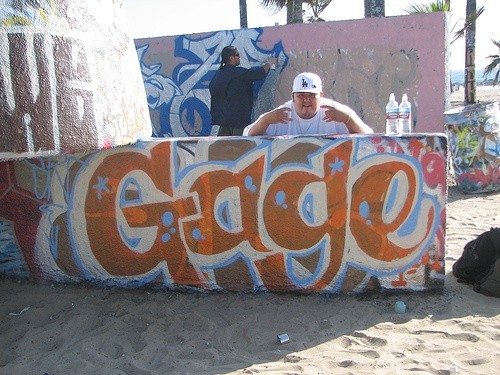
[443,101,500,194]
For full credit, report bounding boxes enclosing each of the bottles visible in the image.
[385,93,399,134]
[398,93,411,134]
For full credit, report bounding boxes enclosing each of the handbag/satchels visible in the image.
[452,227,500,284]
[473,257,500,298]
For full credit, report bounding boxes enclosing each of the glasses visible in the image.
[232,53,239,57]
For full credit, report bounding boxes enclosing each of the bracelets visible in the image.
[344,111,351,124]
[265,61,271,68]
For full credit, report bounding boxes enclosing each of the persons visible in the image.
[209,45,278,136]
[241,72,375,137]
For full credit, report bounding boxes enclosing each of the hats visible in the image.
[292,72,321,95]
